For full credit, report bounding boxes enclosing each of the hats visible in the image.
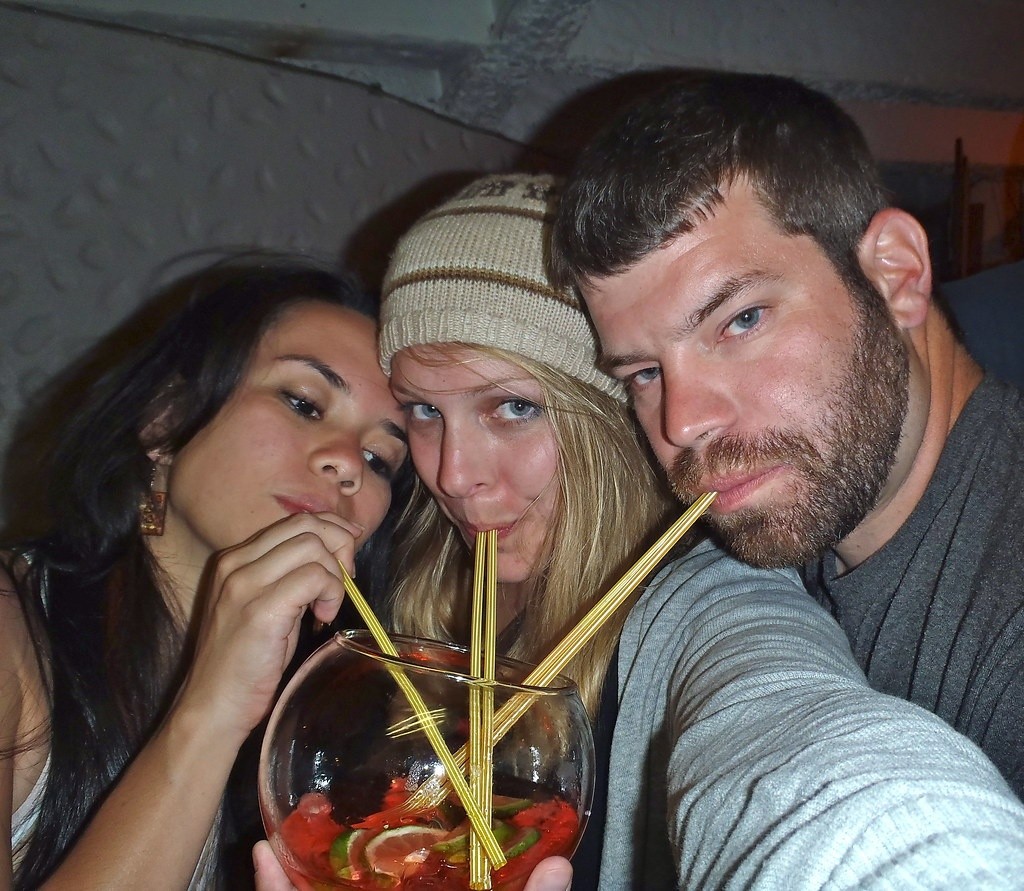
[380,174,633,407]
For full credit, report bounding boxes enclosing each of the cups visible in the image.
[258,628,596,891]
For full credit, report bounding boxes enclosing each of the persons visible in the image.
[0,265,410,891]
[545,72,1024,800]
[252,173,1023,891]
